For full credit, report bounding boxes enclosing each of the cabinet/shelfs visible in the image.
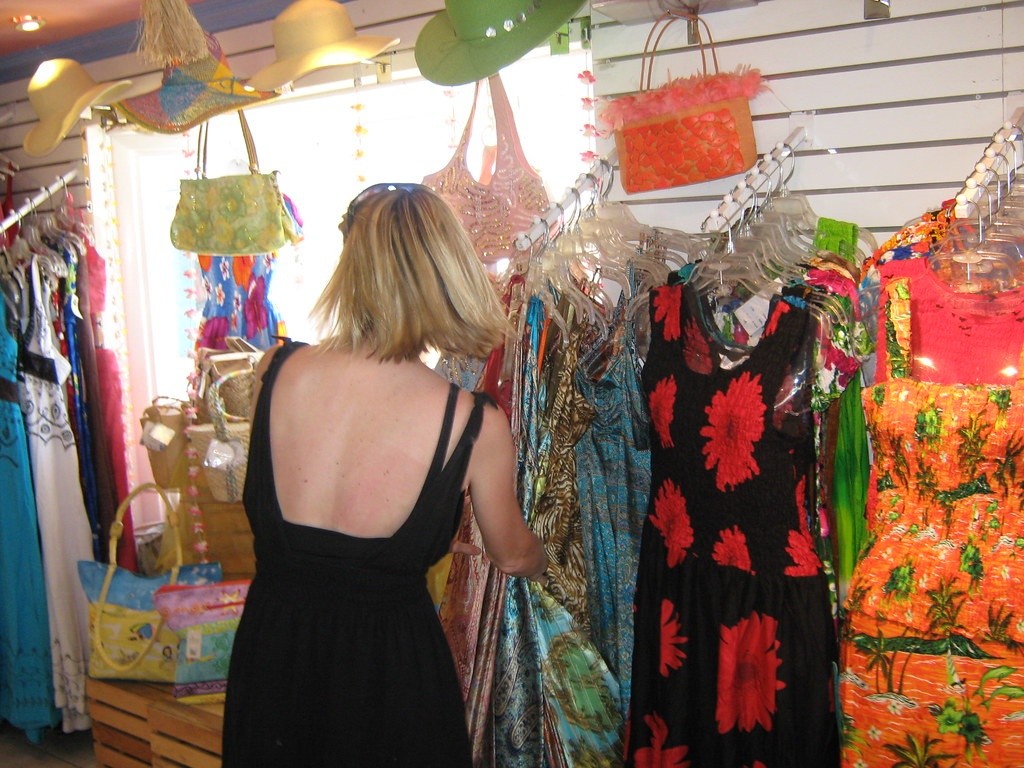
[82,502,255,768]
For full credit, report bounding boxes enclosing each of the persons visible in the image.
[222,182,553,768]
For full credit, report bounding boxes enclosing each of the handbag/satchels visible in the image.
[149,583,256,695]
[74,482,221,683]
[139,336,263,504]
[169,110,301,257]
[607,9,763,193]
[420,76,553,263]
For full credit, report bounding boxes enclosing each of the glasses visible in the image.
[344,182,421,228]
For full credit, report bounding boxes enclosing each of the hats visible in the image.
[245,0,399,92]
[22,59,133,160]
[415,1,587,87]
[113,34,282,133]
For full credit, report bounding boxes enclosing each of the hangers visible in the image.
[0,175,97,323]
[501,118,1024,301]
